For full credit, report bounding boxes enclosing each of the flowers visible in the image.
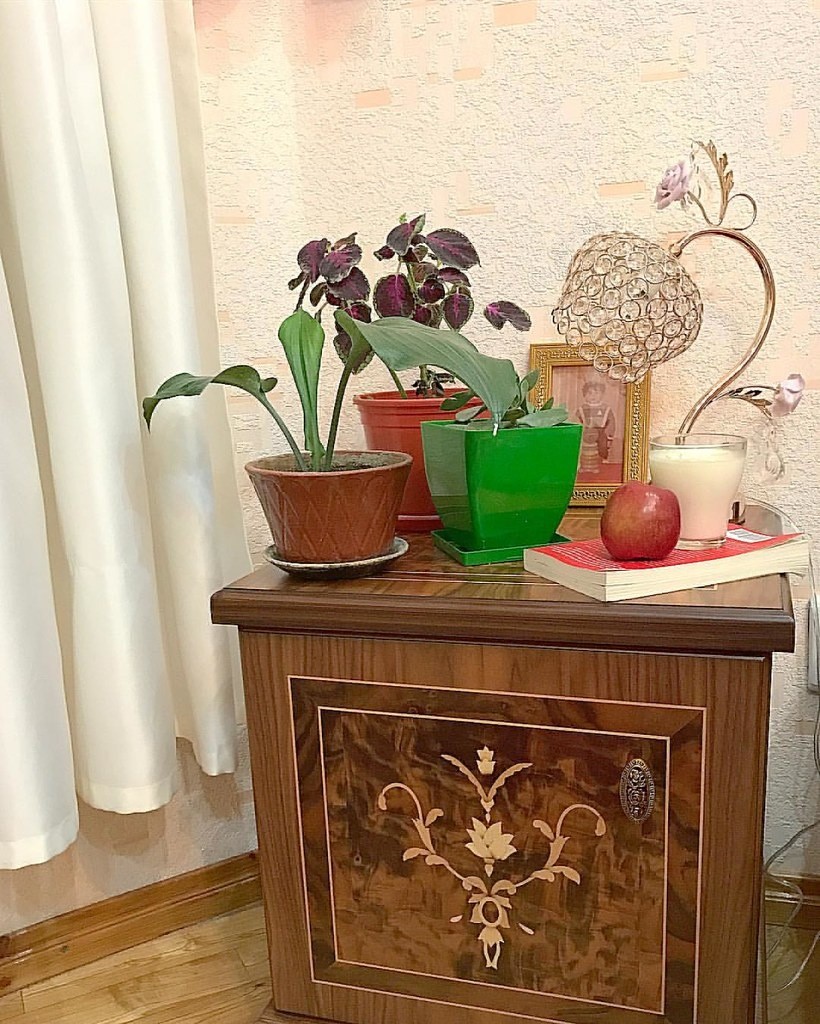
[291,212,533,516]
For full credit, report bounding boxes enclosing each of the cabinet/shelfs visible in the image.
[210,506,795,1024]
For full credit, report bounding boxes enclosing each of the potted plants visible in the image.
[422,375,584,566]
[144,307,517,564]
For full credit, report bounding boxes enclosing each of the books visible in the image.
[524,523,809,603]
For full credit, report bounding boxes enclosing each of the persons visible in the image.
[573,382,615,459]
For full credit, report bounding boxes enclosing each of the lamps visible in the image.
[552,138,805,526]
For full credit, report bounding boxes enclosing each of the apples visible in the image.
[600,480,682,560]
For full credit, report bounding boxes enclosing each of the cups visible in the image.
[648,432,748,551]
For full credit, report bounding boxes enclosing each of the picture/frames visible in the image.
[526,344,650,508]
